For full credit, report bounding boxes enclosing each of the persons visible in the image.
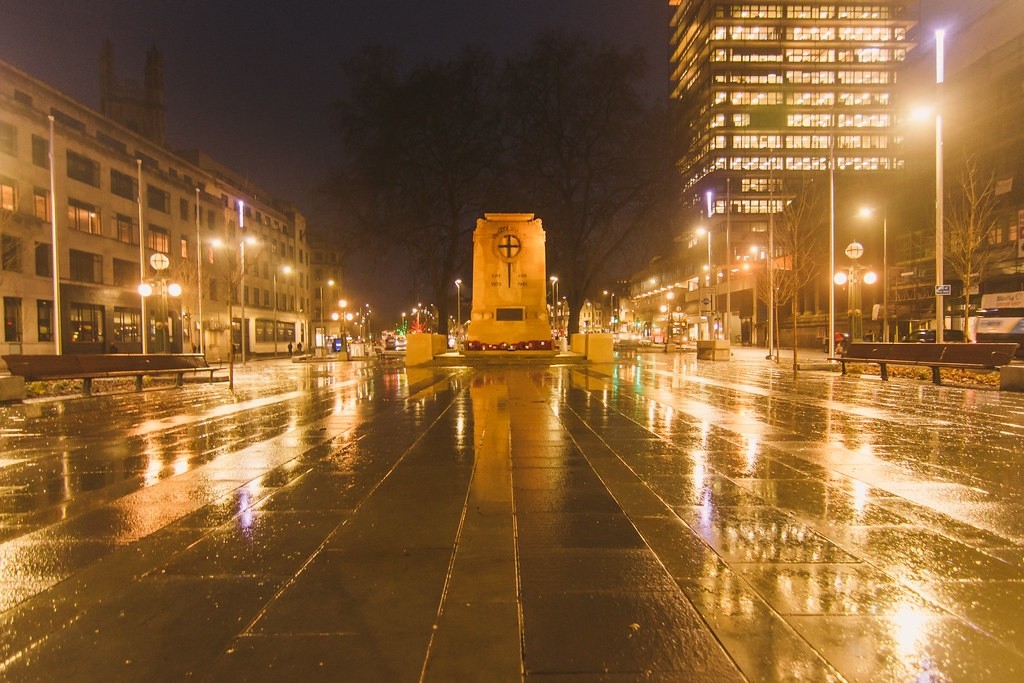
[193,343,205,354]
[865,329,876,342]
[288,341,303,357]
[385,334,395,350]
[555,334,559,340]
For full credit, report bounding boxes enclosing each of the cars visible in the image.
[612,332,653,349]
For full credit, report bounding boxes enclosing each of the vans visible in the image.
[898,329,973,344]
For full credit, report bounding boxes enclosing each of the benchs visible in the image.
[374,348,387,359]
[615,339,639,352]
[2,353,227,397]
[826,342,1017,385]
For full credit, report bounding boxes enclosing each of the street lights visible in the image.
[138,274,182,354]
[833,255,877,344]
[320,280,335,355]
[454,279,462,345]
[550,276,558,351]
[661,291,682,344]
[331,299,353,351]
[273,265,290,356]
